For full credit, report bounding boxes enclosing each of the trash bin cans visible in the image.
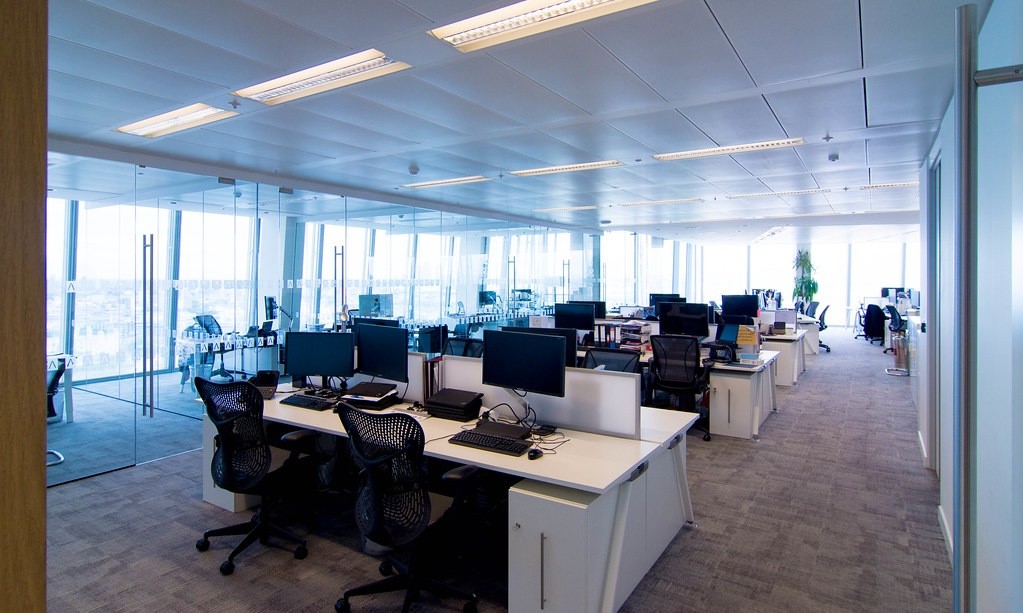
[189,364,212,392]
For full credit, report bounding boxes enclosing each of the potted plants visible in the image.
[792,247,819,313]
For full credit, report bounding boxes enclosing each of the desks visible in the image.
[193,311,819,613]
[885,308,920,321]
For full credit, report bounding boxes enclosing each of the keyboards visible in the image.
[279,393,334,411]
[448,429,533,457]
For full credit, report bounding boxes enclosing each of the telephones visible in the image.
[769,321,785,335]
[243,370,280,400]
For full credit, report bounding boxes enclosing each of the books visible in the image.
[605,320,651,352]
[600,324,606,344]
[594,324,599,344]
[739,353,764,365]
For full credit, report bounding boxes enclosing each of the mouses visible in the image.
[528,449,543,460]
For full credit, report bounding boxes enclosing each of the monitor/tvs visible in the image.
[722,294,759,318]
[910,289,920,307]
[284,332,354,391]
[569,301,606,319]
[649,294,686,317]
[482,330,566,397]
[264,296,277,320]
[357,323,408,383]
[359,294,393,318]
[353,318,399,346]
[658,302,709,337]
[554,304,595,330]
[418,326,448,353]
[502,327,578,367]
[888,289,897,305]
[478,289,532,305]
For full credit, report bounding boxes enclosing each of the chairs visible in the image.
[805,301,831,352]
[854,304,907,353]
[46,358,67,466]
[180,302,716,613]
